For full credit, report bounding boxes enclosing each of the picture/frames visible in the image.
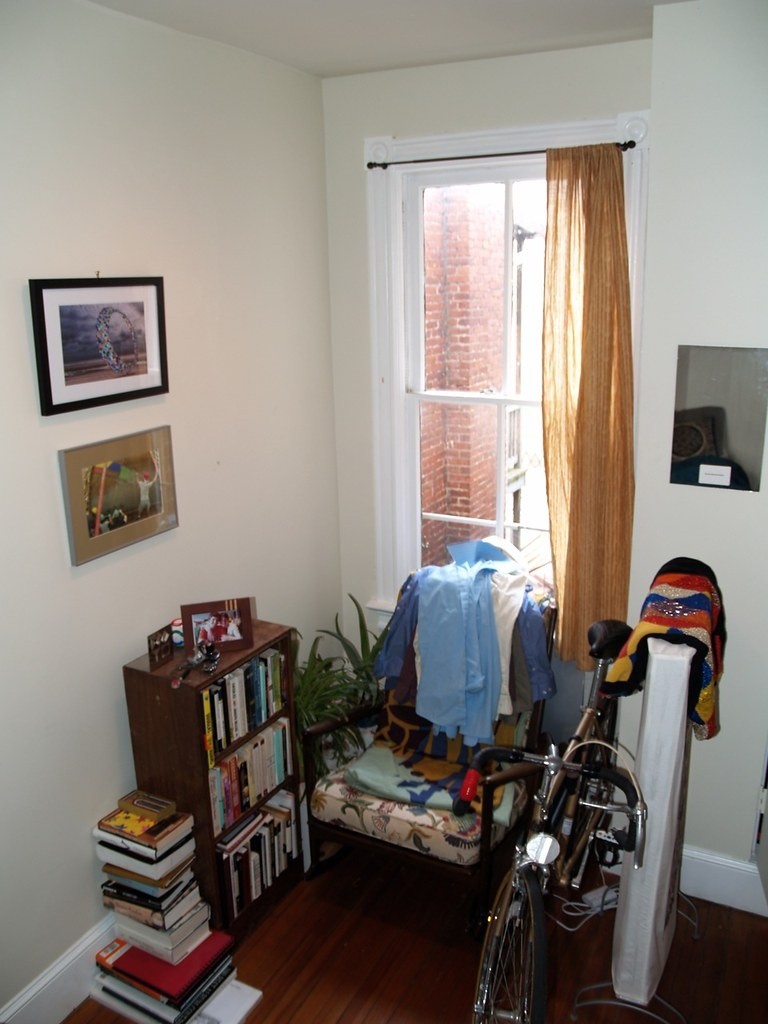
[28,276,172,416]
[58,424,181,567]
[181,597,254,657]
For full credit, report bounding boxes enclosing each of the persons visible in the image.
[196,610,243,646]
[152,628,171,663]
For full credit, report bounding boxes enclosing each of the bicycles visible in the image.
[456,618,648,1024]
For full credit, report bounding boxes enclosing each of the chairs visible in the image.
[303,566,557,941]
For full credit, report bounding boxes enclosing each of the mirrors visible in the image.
[670,344,768,492]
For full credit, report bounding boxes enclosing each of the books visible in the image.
[200,649,312,920]
[90,789,264,1024]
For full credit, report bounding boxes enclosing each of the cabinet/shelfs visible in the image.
[123,618,321,940]
